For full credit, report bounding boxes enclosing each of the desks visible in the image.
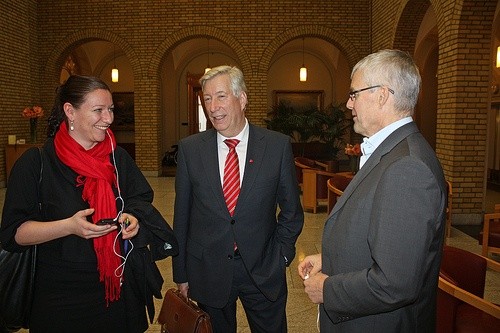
[327,176,354,215]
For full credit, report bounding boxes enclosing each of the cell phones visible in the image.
[95,218,118,227]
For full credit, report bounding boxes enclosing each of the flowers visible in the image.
[21,105,47,142]
[344,143,361,171]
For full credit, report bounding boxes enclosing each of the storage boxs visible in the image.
[8,135,17,145]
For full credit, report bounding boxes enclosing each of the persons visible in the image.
[297,48,447,333]
[0,74,154,333]
[172,66,304,333]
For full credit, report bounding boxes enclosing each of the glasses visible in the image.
[348,83,396,102]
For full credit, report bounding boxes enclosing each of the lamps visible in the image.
[299,37,308,82]
[111,43,120,82]
[205,37,211,75]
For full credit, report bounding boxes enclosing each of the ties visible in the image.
[220,138,241,253]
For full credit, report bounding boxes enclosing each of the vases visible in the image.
[30,117,38,144]
[350,156,359,176]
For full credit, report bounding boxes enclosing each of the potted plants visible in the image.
[312,100,355,173]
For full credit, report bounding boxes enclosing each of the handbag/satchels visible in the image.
[0,245,37,331]
[155,288,213,333]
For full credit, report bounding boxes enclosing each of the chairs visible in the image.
[294,156,329,195]
[446,180,452,238]
[302,168,334,215]
[436,246,500,333]
[479,202,500,273]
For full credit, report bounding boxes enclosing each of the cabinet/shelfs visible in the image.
[5,144,45,188]
